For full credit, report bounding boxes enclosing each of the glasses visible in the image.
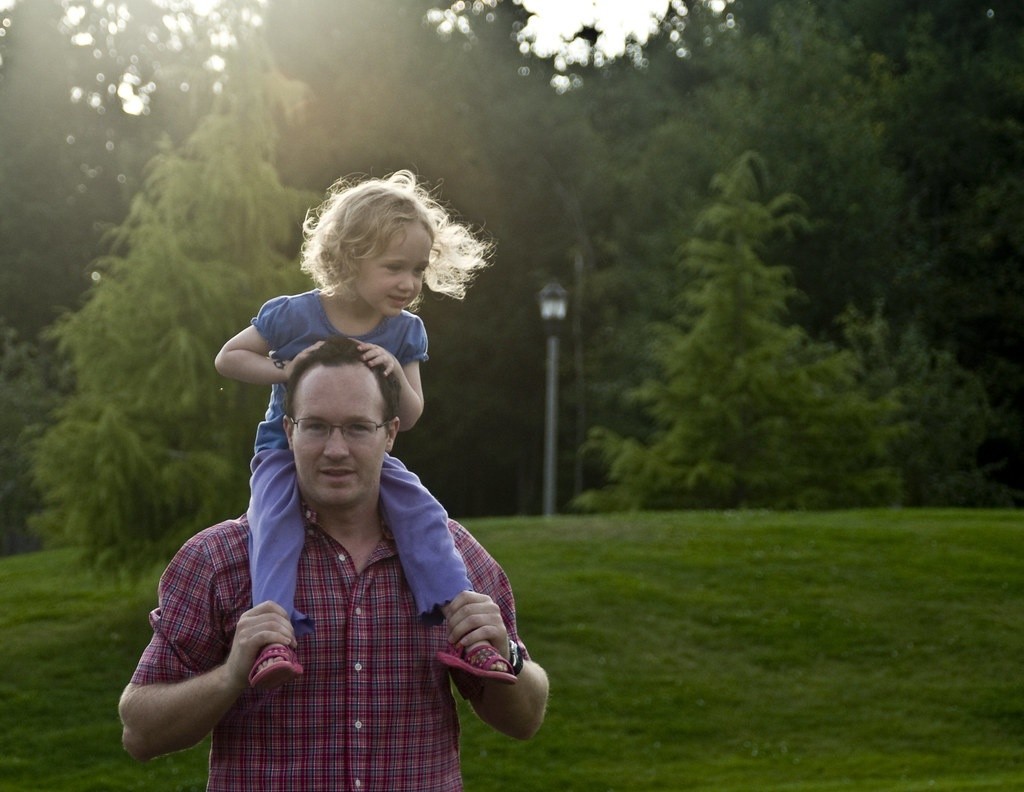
[289,417,391,442]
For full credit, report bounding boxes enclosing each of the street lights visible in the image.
[539,278,569,519]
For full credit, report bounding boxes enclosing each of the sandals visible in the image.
[437,641,519,685]
[249,642,304,690]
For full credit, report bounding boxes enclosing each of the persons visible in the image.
[213,168,520,686]
[118,335,551,792]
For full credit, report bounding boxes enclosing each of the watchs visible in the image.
[509,639,523,676]
